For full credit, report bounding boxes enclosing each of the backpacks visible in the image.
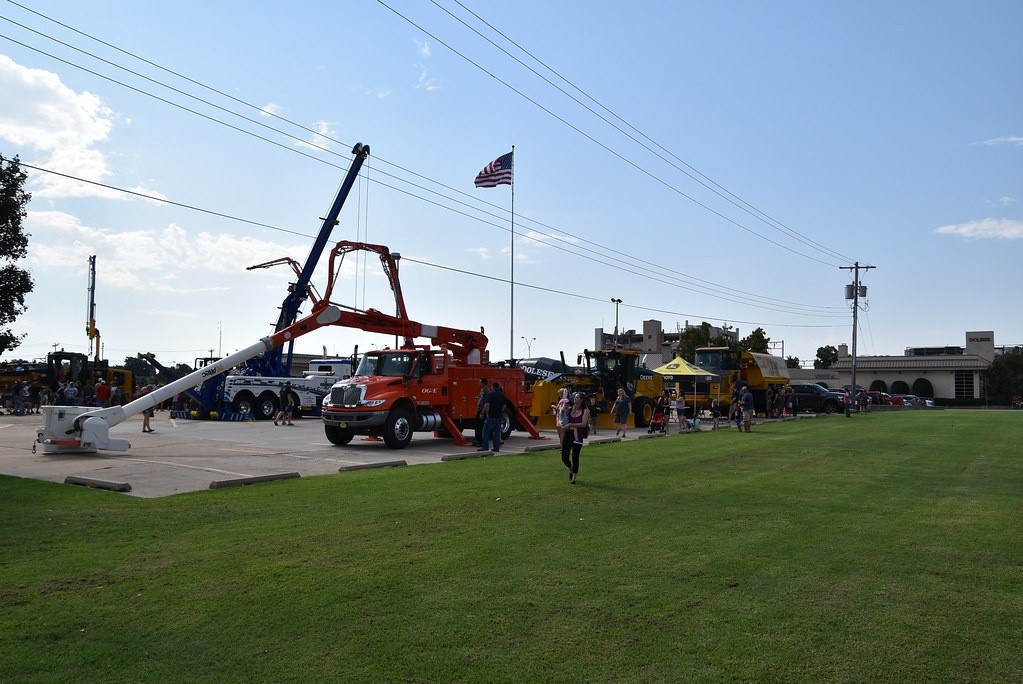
[569,406,590,439]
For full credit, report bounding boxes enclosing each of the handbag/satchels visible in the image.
[285,403,294,413]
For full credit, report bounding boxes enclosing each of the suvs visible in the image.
[787,384,846,413]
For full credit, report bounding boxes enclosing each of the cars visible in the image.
[827,389,932,407]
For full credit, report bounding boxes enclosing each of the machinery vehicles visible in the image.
[137,142,371,420]
[47,254,136,408]
[31,239,550,454]
[530,344,665,429]
[670,347,791,419]
[0,361,47,402]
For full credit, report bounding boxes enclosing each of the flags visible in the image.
[474,152,512,188]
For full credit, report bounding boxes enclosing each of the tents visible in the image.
[651,356,721,417]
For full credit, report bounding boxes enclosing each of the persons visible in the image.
[550,380,633,484]
[655,384,756,433]
[274,381,295,426]
[12,367,188,433]
[766,384,799,418]
[843,386,869,418]
[472,378,507,452]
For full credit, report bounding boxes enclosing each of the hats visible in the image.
[98,378,102,381]
[101,380,106,384]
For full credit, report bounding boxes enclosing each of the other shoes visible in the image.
[571,481,576,484]
[26,411,40,415]
[274,420,278,425]
[282,422,286,425]
[621,434,625,437]
[476,448,489,452]
[148,429,155,432]
[287,423,294,426]
[491,449,499,453]
[472,441,482,447]
[558,450,562,454]
[142,430,150,432]
[615,429,620,436]
[569,473,573,481]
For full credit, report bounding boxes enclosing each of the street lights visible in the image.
[521,336,536,359]
[610,298,622,349]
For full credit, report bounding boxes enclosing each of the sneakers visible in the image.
[574,440,583,444]
